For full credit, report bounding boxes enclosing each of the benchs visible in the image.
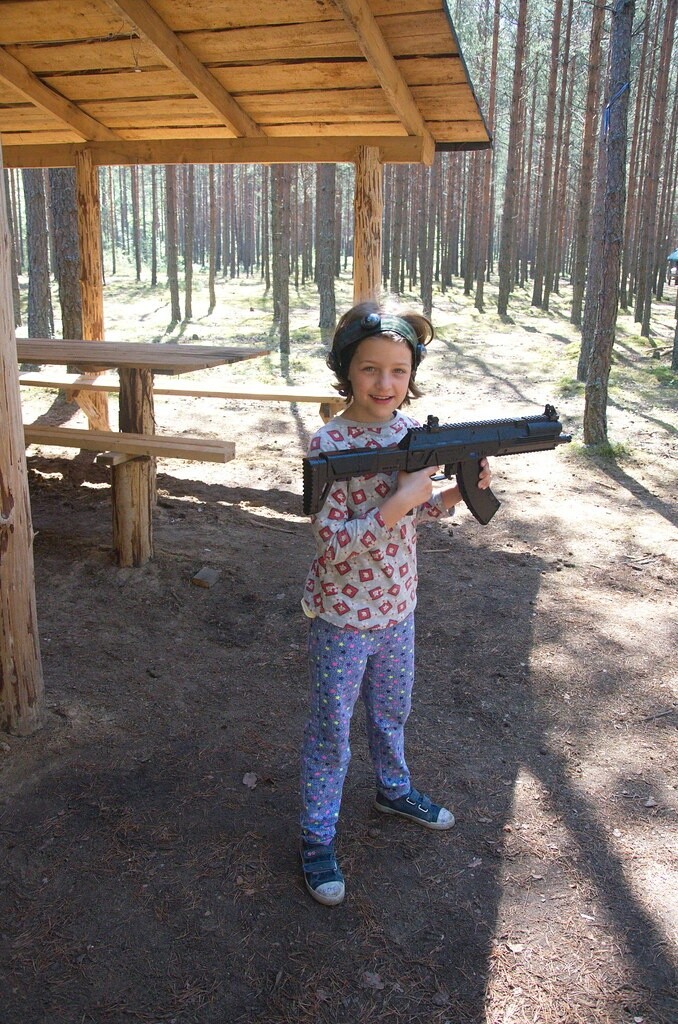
[23,424,235,566]
[17,369,349,431]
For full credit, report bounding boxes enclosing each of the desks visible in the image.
[15,337,273,567]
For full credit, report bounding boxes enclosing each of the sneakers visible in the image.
[375,787,455,830]
[298,837,345,905]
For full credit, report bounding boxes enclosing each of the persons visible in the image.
[299,302,494,905]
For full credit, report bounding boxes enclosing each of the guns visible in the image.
[301,401,572,525]
